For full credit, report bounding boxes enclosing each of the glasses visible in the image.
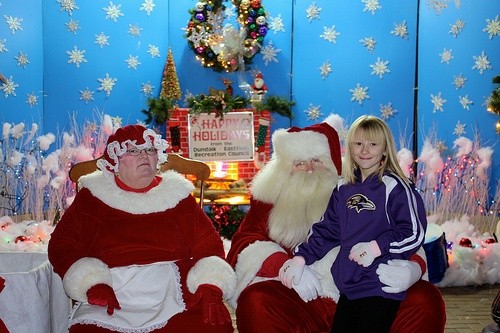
[126,147,157,156]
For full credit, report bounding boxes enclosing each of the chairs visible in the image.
[69,152,211,208]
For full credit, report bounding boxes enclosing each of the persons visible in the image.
[226,114,447,333]
[47,124,237,333]
[278,115,429,333]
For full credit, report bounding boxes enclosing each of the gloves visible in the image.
[279,257,305,289]
[280,258,324,302]
[185,283,231,325]
[376,258,422,294]
[86,283,122,316]
[348,240,381,267]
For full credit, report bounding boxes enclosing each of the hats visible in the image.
[96,125,169,174]
[272,113,343,177]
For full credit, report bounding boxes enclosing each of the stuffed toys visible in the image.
[251,73,268,96]
